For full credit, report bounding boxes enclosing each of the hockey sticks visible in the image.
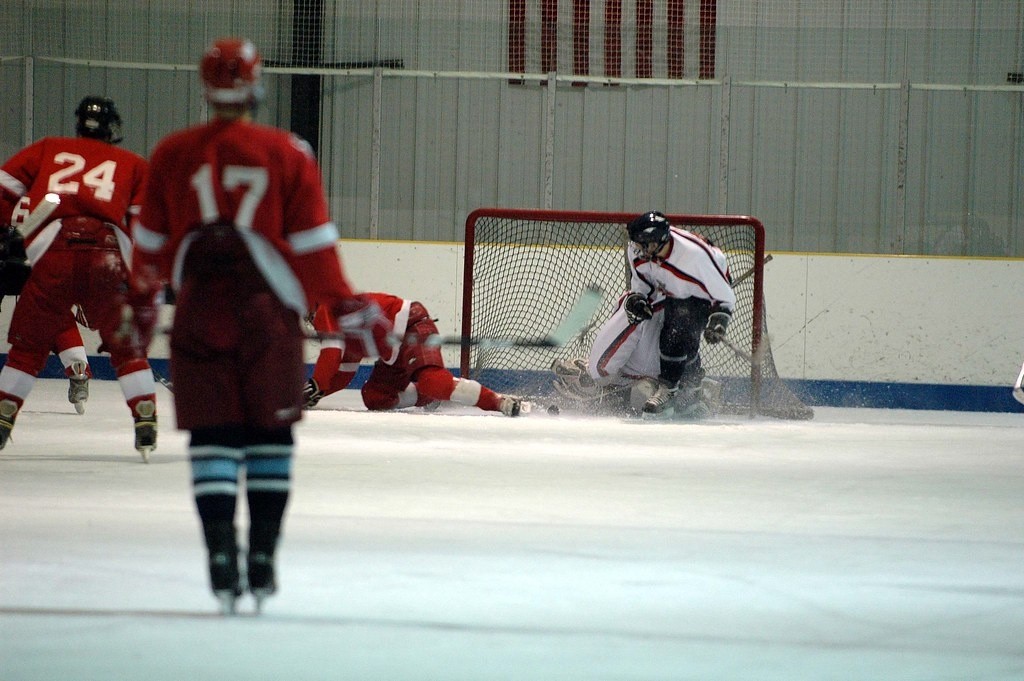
[551,254,773,401]
[299,284,602,352]
[154,363,321,414]
[708,327,815,420]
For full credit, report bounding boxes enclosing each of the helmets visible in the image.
[298,309,322,340]
[74,94,123,144]
[628,211,670,260]
[199,38,264,111]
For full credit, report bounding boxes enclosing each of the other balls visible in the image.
[547,406,560,417]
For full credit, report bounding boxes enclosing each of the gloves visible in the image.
[704,306,732,344]
[301,377,324,408]
[0,224,27,262]
[128,288,157,347]
[624,292,653,325]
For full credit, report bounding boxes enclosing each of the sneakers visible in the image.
[67,361,90,414]
[642,374,680,420]
[497,394,532,417]
[247,551,275,615]
[210,552,243,616]
[133,400,158,465]
[672,369,706,416]
[0,399,18,450]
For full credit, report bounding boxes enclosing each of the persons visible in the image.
[116,39,373,613]
[0,95,159,462]
[301,293,531,416]
[551,211,737,420]
[0,188,92,415]
